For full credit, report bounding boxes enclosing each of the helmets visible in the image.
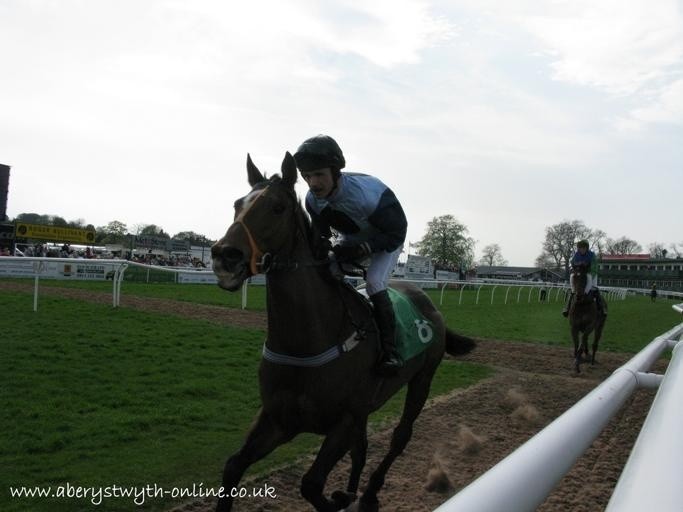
[294,134,345,170]
[577,239,590,245]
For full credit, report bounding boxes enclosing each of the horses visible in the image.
[567,268,609,374]
[650,290,657,301]
[209,150,481,512]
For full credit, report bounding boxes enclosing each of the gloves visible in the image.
[347,242,371,263]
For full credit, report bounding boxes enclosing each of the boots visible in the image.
[562,292,572,317]
[369,288,403,370]
[598,300,607,318]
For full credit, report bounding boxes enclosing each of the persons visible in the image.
[562,240,608,318]
[540,287,546,300]
[293,134,407,377]
